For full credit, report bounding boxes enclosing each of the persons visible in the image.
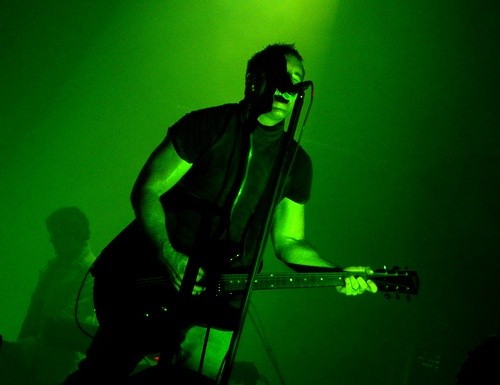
[55,41,378,385]
[18,204,99,338]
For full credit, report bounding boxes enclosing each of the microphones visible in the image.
[288,80,311,96]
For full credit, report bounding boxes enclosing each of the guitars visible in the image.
[95,240,419,355]
